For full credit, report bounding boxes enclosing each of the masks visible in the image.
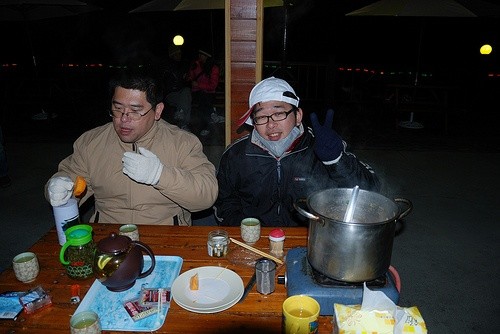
[254,127,300,157]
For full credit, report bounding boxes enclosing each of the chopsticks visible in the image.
[230,237,282,264]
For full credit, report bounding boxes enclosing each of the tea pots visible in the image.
[93,233,156,292]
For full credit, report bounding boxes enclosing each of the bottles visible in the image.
[207,229,230,257]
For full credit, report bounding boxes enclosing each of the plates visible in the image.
[171,266,244,314]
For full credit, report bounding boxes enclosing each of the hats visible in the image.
[237,75,299,126]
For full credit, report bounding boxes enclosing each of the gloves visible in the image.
[122,147,163,186]
[47,176,74,206]
[311,109,343,165]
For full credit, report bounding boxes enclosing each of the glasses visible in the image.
[252,108,294,125]
[109,105,153,121]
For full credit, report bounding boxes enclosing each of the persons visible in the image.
[44,73,219,226]
[214,77,382,227]
[162,44,191,125]
[186,49,219,145]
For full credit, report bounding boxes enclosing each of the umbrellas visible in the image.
[344,0,479,120]
[127,0,296,66]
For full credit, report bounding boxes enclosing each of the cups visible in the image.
[241,217,261,244]
[255,259,277,296]
[12,252,39,284]
[70,309,102,334]
[119,224,139,242]
[59,224,97,280]
[282,295,320,334]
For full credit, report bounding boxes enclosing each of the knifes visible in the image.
[238,273,256,304]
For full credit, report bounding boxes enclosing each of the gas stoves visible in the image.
[277,247,400,315]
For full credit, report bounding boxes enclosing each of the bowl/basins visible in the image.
[293,188,412,283]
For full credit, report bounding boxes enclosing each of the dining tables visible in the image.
[0,219,336,334]
